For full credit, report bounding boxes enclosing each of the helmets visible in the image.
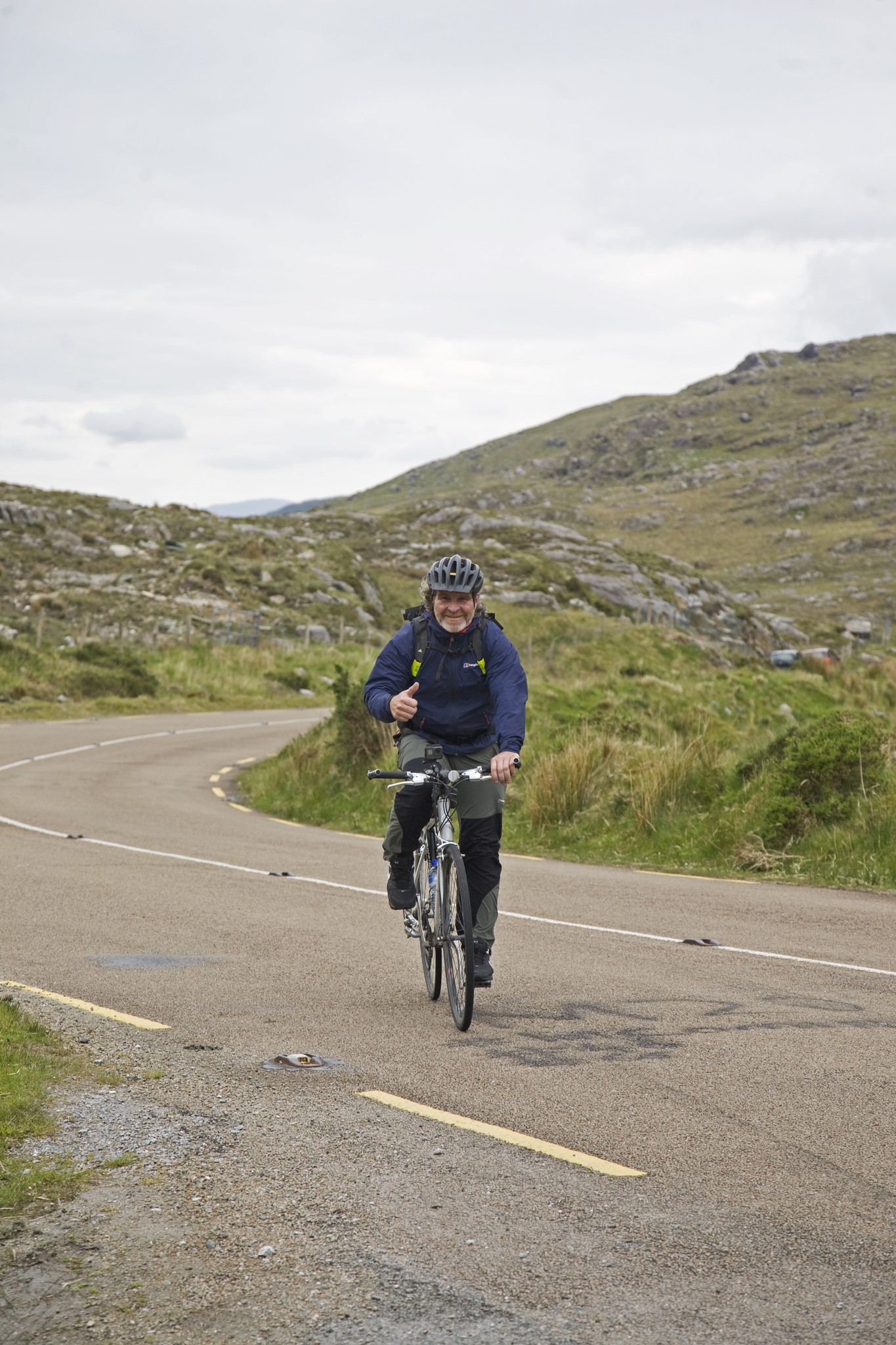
[426,553,484,593]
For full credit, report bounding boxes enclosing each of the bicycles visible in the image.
[367,743,522,1032]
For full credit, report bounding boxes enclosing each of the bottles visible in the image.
[428,858,438,912]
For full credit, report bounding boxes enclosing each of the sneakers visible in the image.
[462,937,493,982]
[386,854,417,910]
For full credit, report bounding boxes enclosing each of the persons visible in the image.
[364,554,528,981]
